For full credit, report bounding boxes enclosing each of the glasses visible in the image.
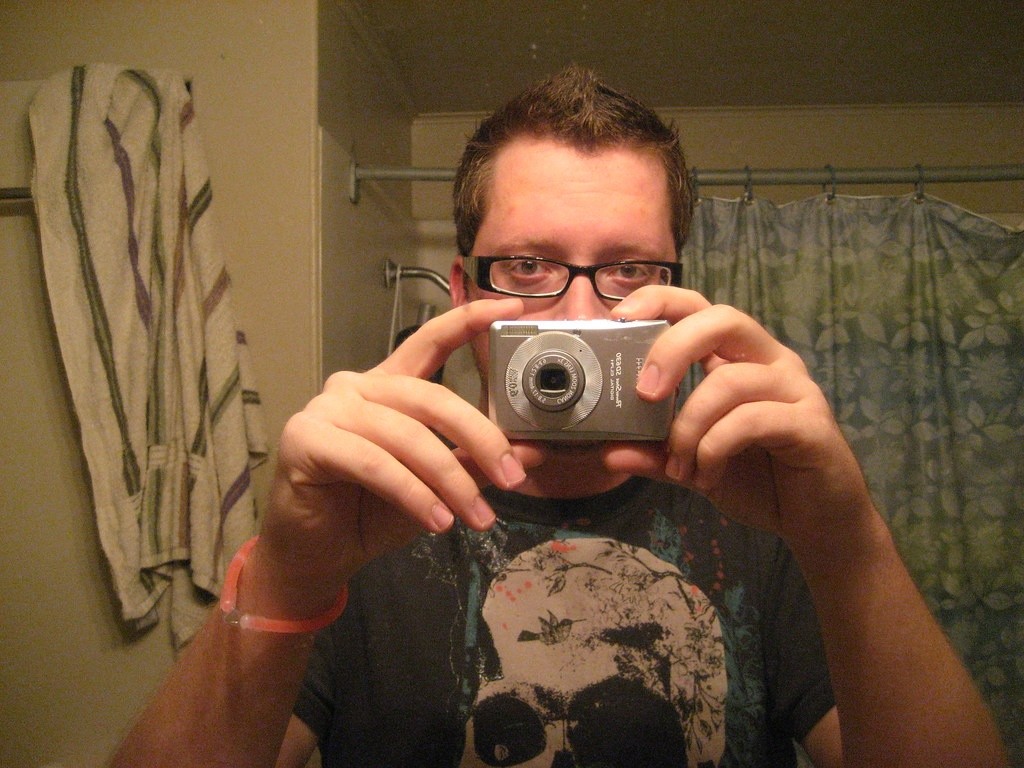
[462,255,683,302]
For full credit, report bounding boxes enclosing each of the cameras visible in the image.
[488,318,676,442]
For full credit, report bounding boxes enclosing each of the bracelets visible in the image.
[219,534,349,632]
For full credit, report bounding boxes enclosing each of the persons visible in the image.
[106,62,1008,768]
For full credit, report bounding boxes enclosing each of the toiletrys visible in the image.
[392,302,445,385]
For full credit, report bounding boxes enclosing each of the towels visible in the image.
[25,62,270,667]
